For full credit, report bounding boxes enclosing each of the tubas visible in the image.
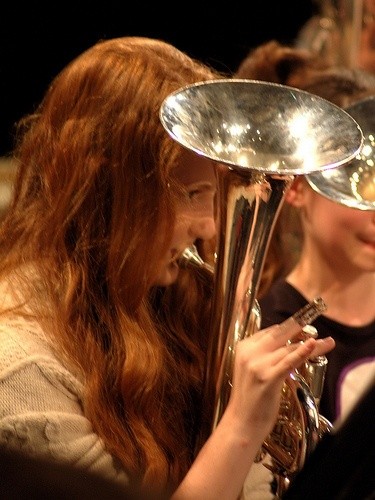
[159,80,364,499]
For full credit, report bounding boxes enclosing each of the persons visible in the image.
[1,37,336,500]
[260,98,374,430]
[296,72,369,109]
[234,42,322,89]
